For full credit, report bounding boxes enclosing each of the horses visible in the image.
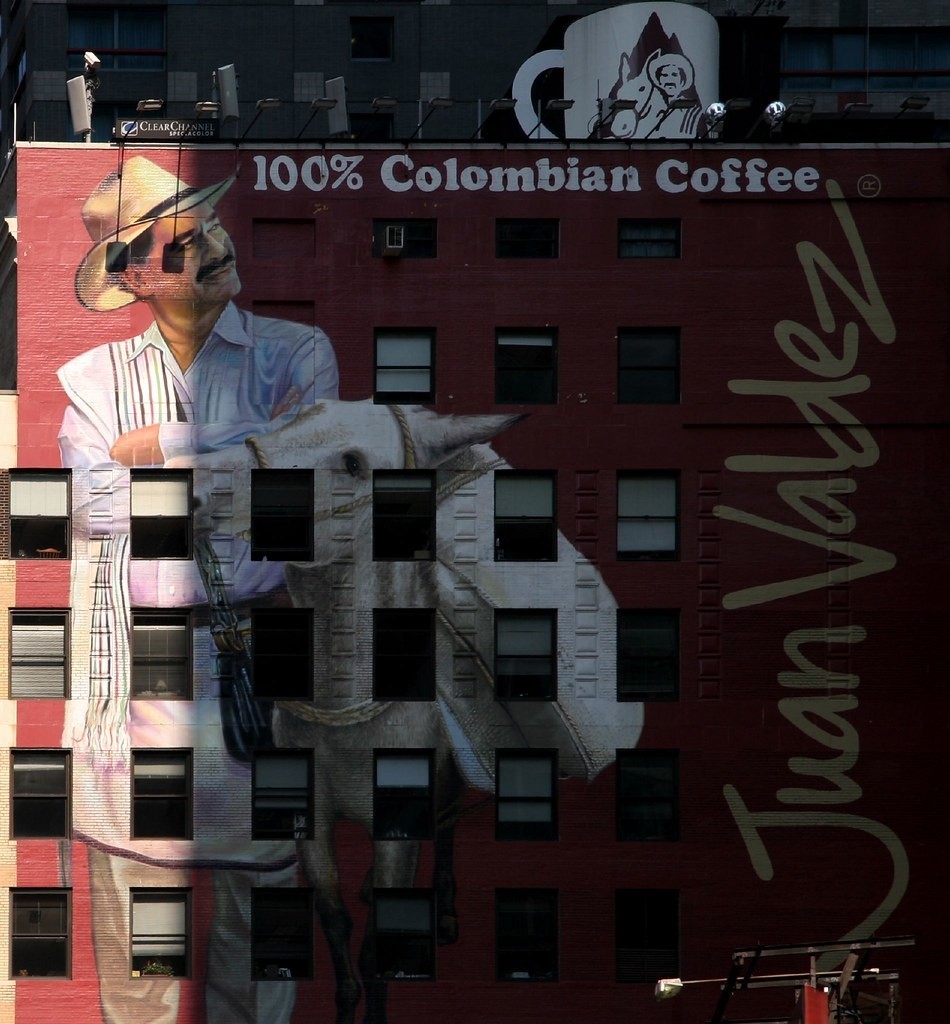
[161,393,647,805]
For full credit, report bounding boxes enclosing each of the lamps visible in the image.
[122,98,163,141]
[297,98,338,138]
[242,98,282,138]
[768,97,815,133]
[700,97,752,139]
[527,100,574,137]
[410,97,455,139]
[644,100,697,140]
[839,102,873,120]
[356,96,399,138]
[182,102,220,139]
[470,99,517,139]
[888,95,929,120]
[588,100,638,139]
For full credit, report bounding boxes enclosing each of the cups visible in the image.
[513,1,722,138]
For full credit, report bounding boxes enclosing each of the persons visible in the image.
[59,158,340,1023]
